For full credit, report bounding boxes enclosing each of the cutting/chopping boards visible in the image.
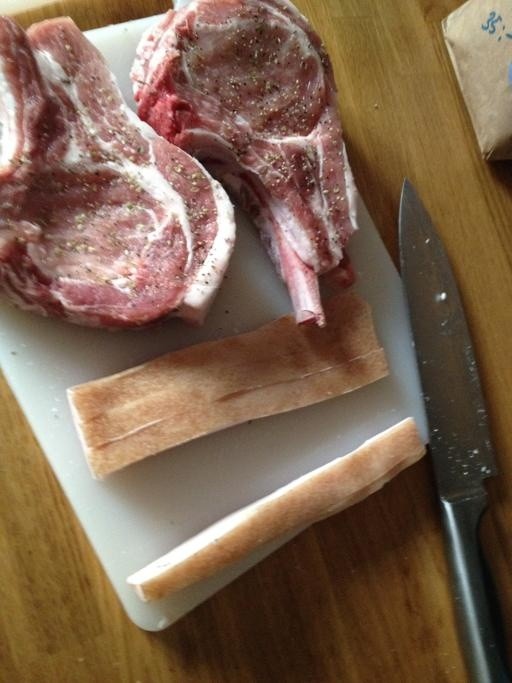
[0,12,428,633]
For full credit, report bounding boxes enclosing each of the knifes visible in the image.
[397,176,512,682]
